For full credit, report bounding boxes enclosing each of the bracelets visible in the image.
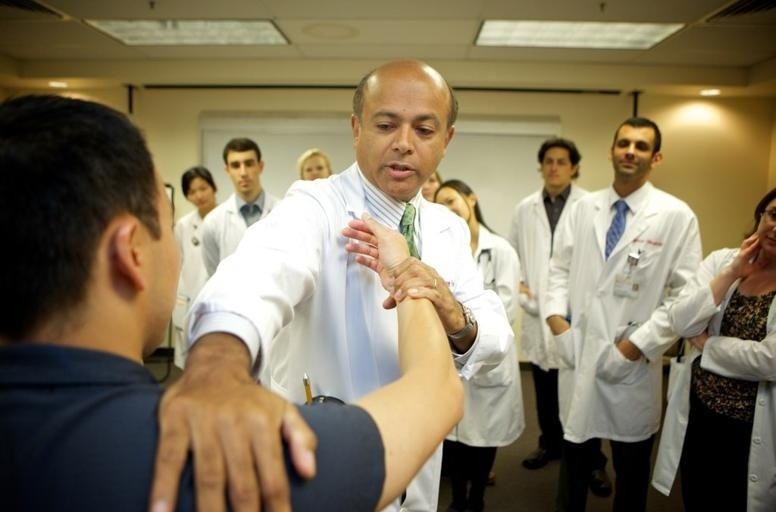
[445,301,475,341]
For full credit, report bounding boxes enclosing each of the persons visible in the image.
[295,148,332,180]
[650,188,776,511]
[541,116,703,511]
[504,134,609,471]
[200,137,286,274]
[167,166,223,372]
[0,93,466,510]
[421,169,440,203]
[145,57,516,510]
[432,179,523,512]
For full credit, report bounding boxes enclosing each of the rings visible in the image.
[434,278,437,289]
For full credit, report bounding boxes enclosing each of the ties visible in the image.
[604,199,630,262]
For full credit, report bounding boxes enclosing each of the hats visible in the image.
[400,204,422,261]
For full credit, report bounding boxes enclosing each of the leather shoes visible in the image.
[522,444,560,471]
[585,464,613,499]
